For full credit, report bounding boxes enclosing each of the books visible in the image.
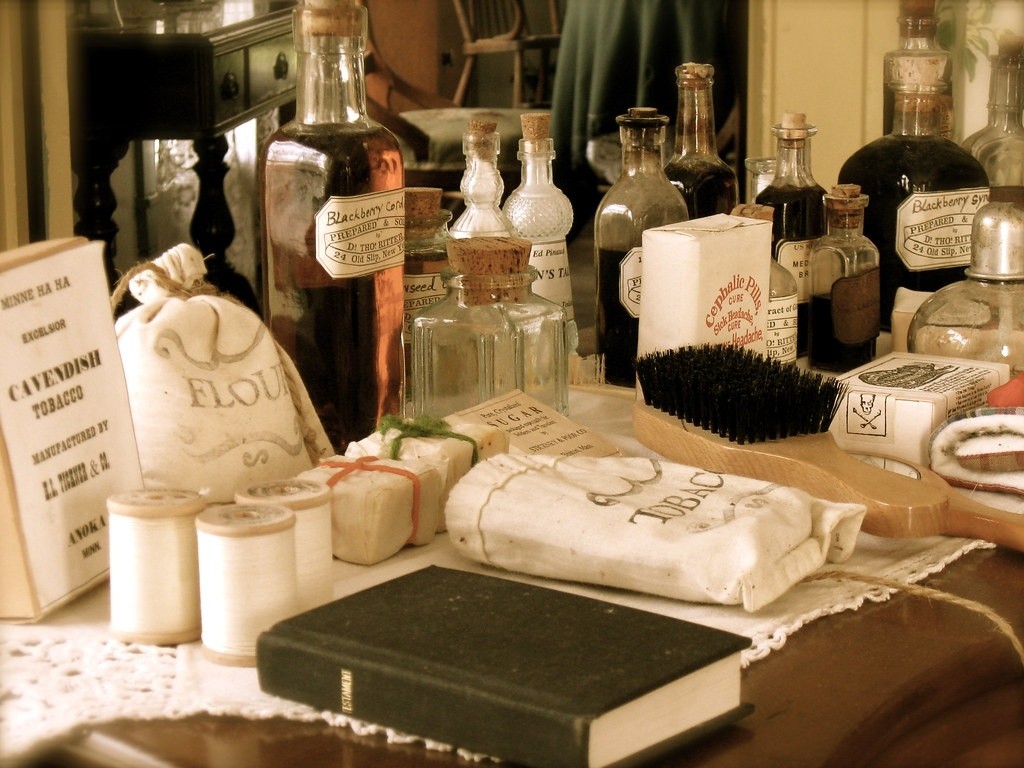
[256,563,756,768]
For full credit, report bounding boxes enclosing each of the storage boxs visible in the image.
[300,456,442,565]
[458,390,626,458]
[368,419,511,531]
[634,211,774,400]
[834,352,1010,478]
[0,236,147,624]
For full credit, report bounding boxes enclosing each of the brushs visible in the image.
[631,343,946,538]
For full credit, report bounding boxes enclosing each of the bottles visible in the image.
[262,0,405,449]
[664,63,738,224]
[404,188,453,408]
[414,237,570,423]
[593,107,689,387]
[734,204,798,373]
[809,184,881,371]
[503,112,579,364]
[970,36,1024,186]
[906,203,1024,376]
[839,0,992,331]
[448,121,516,237]
[745,158,777,201]
[754,112,828,354]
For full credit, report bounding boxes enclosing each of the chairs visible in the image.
[360,0,562,163]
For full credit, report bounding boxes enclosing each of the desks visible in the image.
[73,2,372,291]
[0,535,1024,768]
